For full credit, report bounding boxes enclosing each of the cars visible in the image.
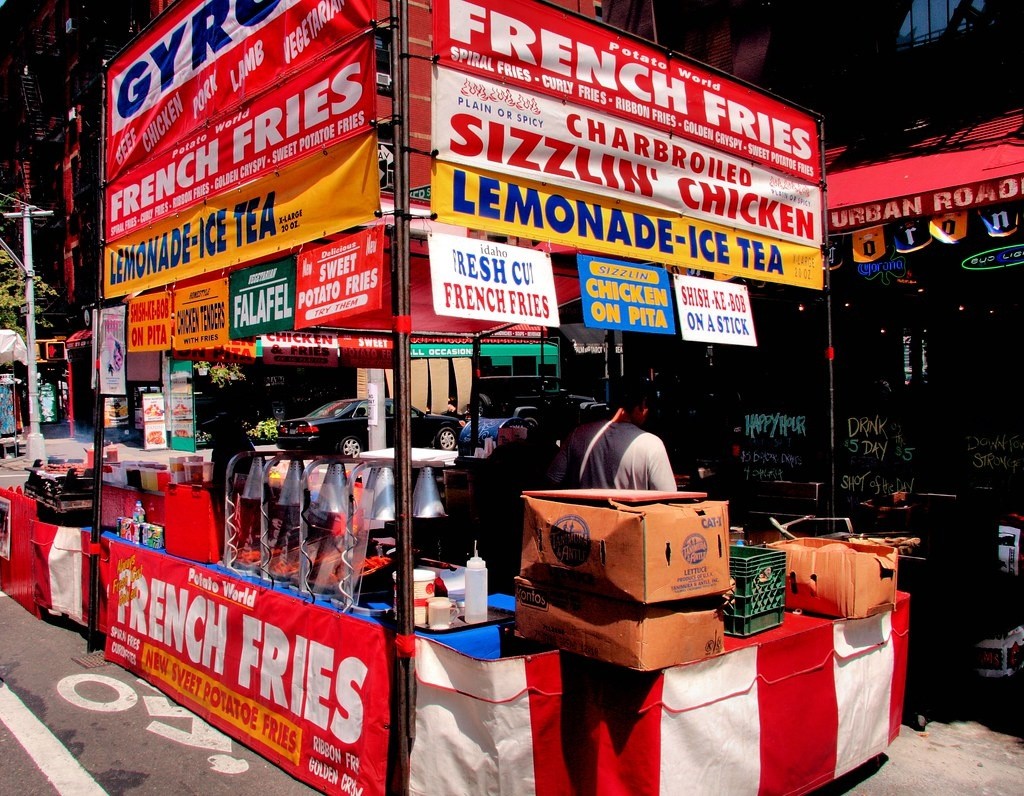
[276,398,463,458]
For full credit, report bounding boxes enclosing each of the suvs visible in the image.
[476,376,561,418]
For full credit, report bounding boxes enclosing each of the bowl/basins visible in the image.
[183,462,214,483]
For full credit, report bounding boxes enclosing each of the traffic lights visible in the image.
[47,340,66,360]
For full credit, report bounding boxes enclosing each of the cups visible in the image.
[425,597,460,630]
[475,448,486,458]
[111,466,158,492]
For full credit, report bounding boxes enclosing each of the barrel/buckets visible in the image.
[392,569,435,625]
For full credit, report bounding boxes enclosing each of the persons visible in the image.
[447,397,457,413]
[479,410,571,596]
[533,373,678,491]
[200,410,255,490]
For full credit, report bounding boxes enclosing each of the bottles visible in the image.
[484,435,493,456]
[106,442,118,462]
[464,549,488,625]
[87,445,94,468]
[132,500,145,545]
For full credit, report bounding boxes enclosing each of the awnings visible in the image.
[822,107,1024,230]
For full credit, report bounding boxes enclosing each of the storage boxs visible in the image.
[520,495,733,604]
[164,481,227,565]
[765,536,899,619]
[514,575,724,672]
[722,546,786,637]
